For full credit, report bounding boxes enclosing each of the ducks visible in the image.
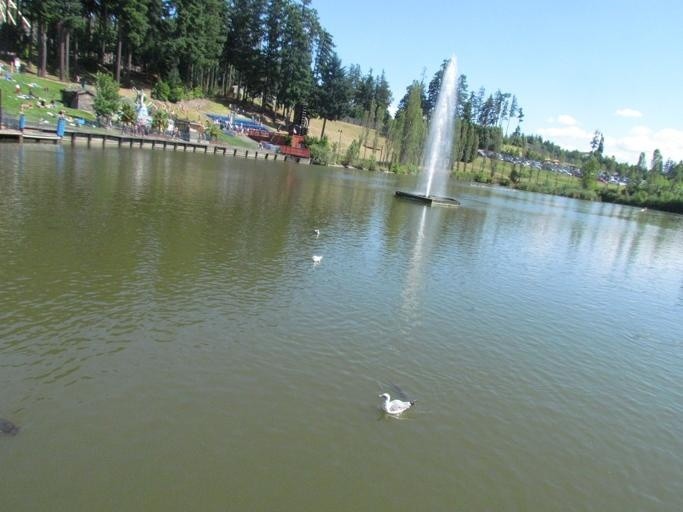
[311,255,323,263]
[313,229,319,235]
[377,392,414,414]
